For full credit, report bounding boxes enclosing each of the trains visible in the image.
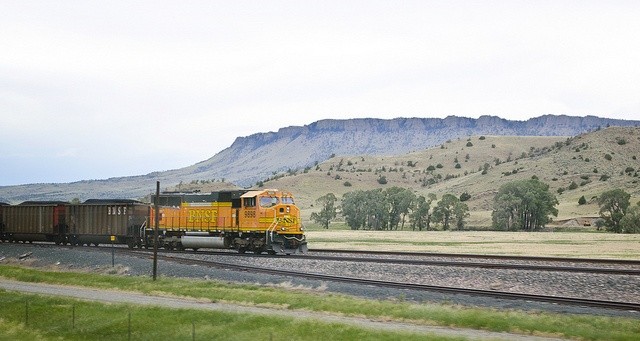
[1,189,308,255]
